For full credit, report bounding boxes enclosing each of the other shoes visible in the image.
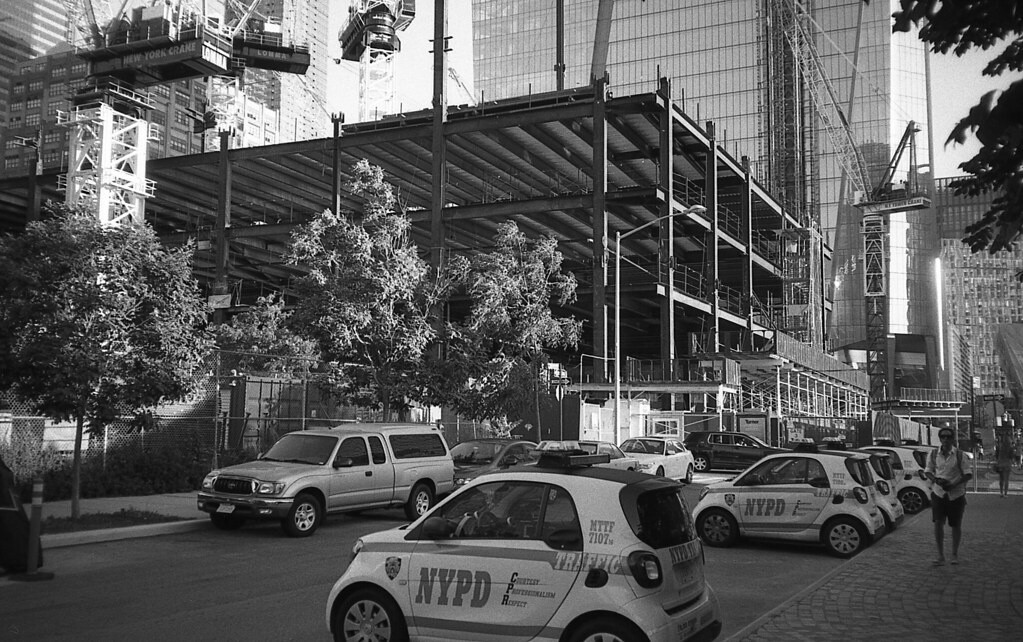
[950,555,959,565]
[932,557,945,566]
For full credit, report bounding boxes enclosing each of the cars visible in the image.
[324,466,723,642]
[622,437,695,483]
[450,438,541,494]
[537,440,640,472]
[692,446,887,558]
[774,446,939,529]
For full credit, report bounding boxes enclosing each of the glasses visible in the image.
[939,435,952,440]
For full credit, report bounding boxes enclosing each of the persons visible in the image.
[736,438,745,446]
[806,463,829,488]
[1015,446,1022,468]
[996,441,1012,497]
[978,446,983,459]
[924,428,973,566]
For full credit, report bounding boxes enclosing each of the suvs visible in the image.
[683,430,793,472]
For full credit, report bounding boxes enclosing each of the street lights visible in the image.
[613,206,701,447]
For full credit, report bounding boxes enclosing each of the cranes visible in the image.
[774,0,933,402]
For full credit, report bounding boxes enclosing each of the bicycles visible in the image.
[987,454,1023,475]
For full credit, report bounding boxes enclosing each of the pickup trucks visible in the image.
[195,422,455,538]
